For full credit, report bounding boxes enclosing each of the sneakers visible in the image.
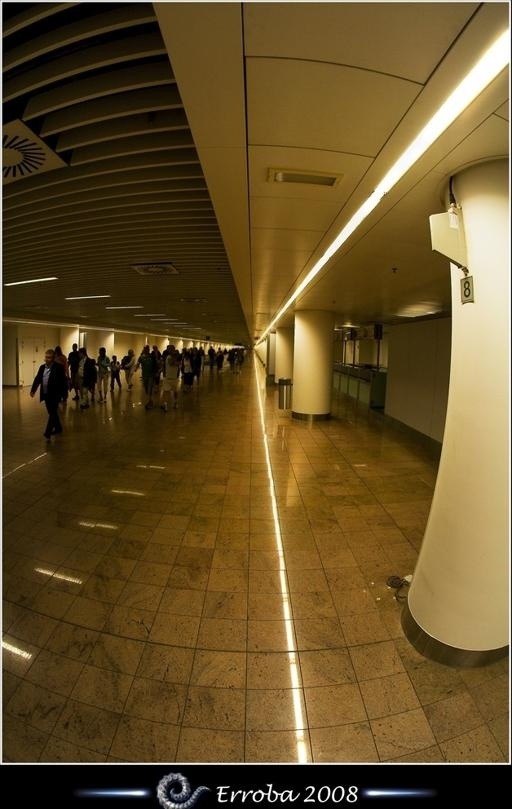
[80,404,89,408]
[98,399,107,404]
[72,396,79,400]
[145,400,178,411]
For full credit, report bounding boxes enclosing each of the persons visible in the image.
[28,348,69,441]
[54,343,248,413]
